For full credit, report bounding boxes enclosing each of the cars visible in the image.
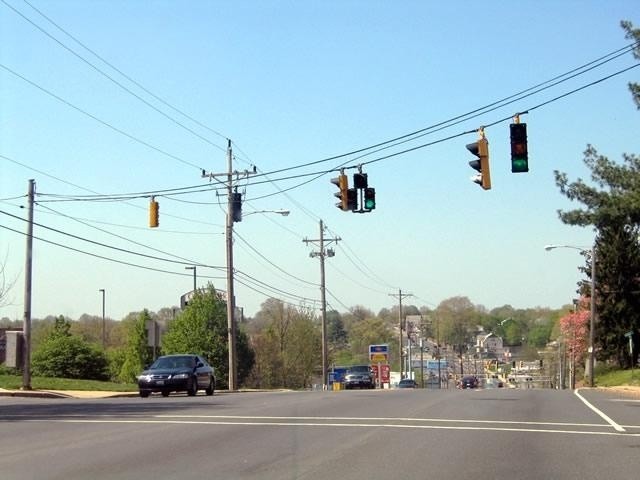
[398,379,419,388]
[460,375,479,389]
[486,375,503,388]
[425,345,449,359]
[138,353,217,398]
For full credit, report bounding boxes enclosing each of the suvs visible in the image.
[344,363,375,389]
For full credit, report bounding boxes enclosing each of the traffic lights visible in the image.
[148,201,160,227]
[363,188,376,210]
[510,123,529,173]
[347,188,359,210]
[466,137,491,190]
[330,174,348,212]
[354,172,367,189]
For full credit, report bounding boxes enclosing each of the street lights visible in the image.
[545,244,597,386]
[226,206,292,390]
[99,289,106,345]
[184,267,197,292]
